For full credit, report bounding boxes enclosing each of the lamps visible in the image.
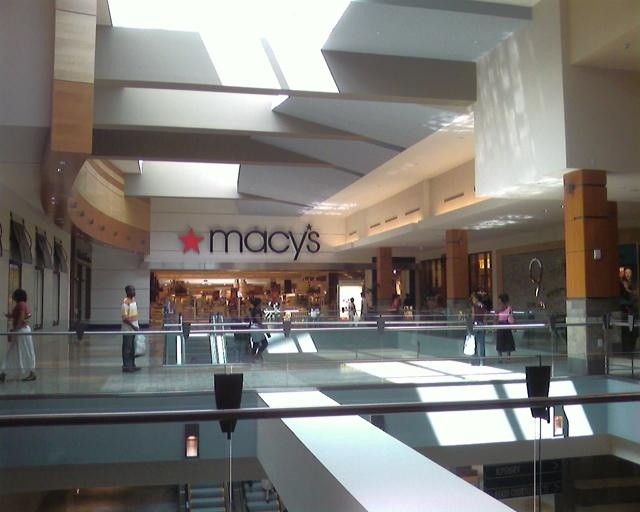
[554,405,569,439]
[370,415,384,431]
[185,424,199,460]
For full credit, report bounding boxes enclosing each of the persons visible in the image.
[250,294,271,362]
[493,292,517,363]
[349,297,358,321]
[0,289,39,382]
[360,292,367,320]
[468,293,486,366]
[121,284,144,372]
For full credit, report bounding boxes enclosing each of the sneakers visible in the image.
[469,359,485,367]
[0,372,6,382]
[22,371,36,382]
[495,359,511,365]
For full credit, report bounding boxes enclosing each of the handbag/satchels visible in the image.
[23,312,33,322]
[463,333,477,356]
[508,315,515,324]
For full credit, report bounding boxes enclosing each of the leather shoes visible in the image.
[122,365,140,373]
